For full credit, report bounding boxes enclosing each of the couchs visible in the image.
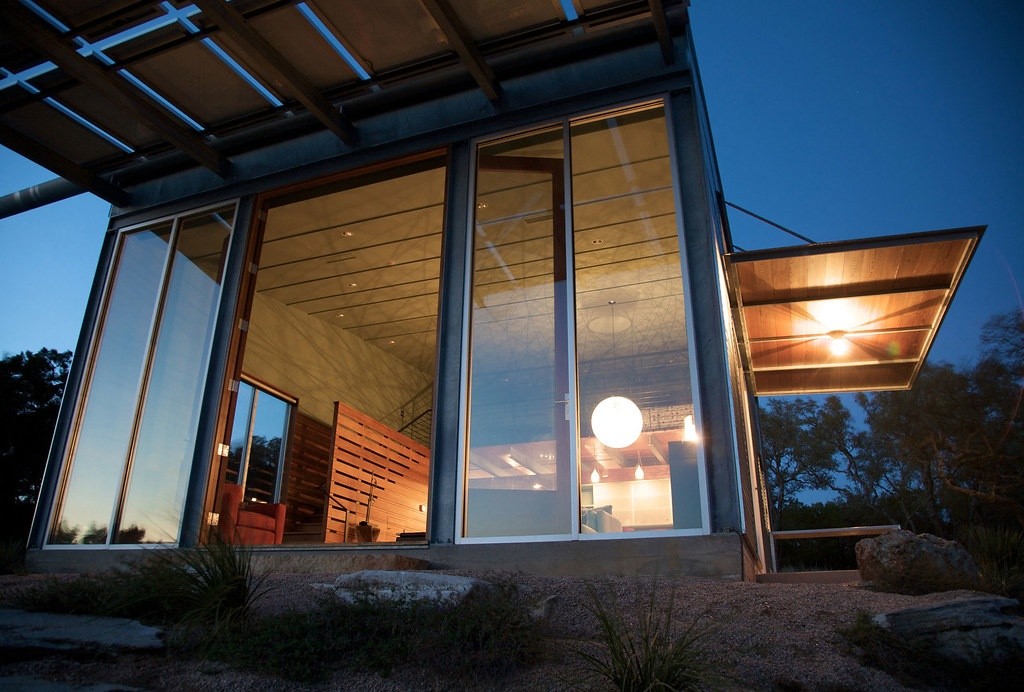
[582,510,622,534]
[217,482,287,545]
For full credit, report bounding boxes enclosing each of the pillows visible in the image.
[586,510,597,530]
[589,505,613,515]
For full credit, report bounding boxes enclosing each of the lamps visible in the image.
[590,439,600,483]
[635,436,644,479]
[590,300,644,449]
